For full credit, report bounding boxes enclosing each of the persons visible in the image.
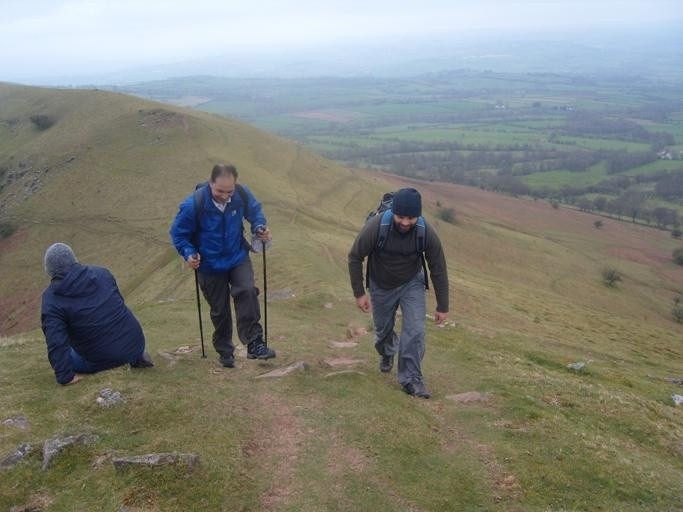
[347,188,448,399]
[39,242,155,386]
[167,163,276,368]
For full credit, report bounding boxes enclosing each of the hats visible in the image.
[44,242,79,276]
[391,187,423,218]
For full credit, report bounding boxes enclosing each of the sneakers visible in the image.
[402,379,430,400]
[129,351,155,369]
[379,352,395,373]
[218,351,235,368]
[246,340,277,359]
